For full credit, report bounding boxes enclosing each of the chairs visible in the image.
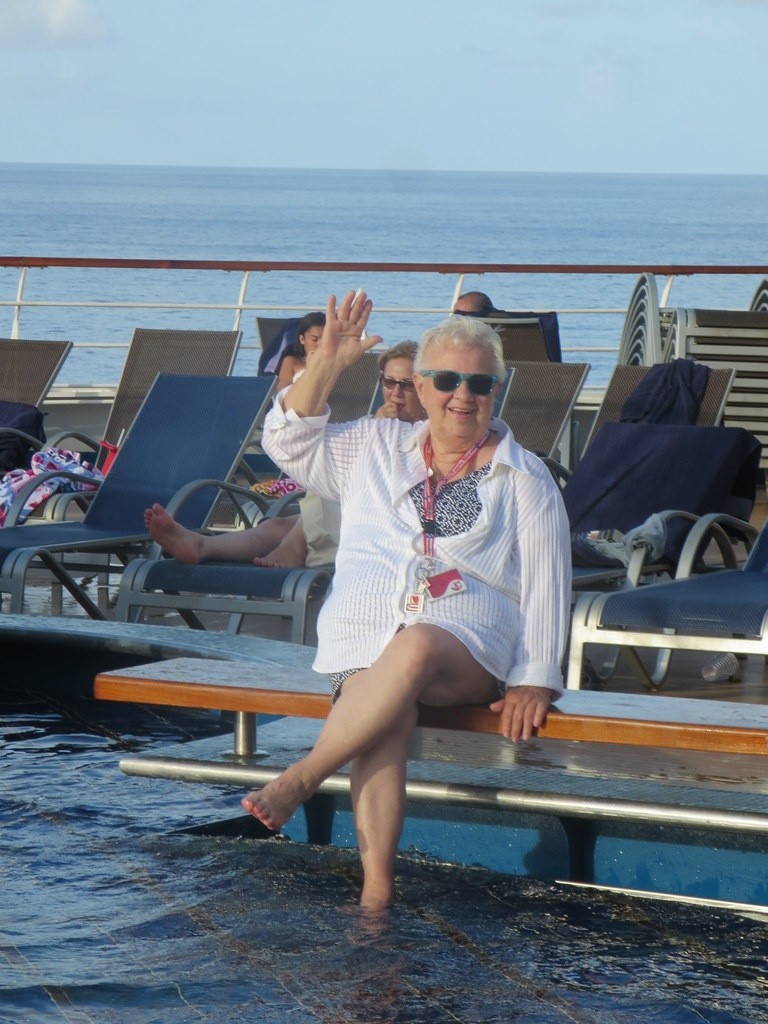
[0,273,768,690]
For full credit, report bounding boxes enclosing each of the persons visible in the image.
[278,312,327,391]
[240,291,573,907]
[455,292,492,316]
[143,341,425,570]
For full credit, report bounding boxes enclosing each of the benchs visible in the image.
[94,657,768,763]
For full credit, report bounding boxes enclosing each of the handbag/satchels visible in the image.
[299,494,340,566]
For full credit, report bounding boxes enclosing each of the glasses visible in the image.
[379,373,415,391]
[418,370,498,395]
